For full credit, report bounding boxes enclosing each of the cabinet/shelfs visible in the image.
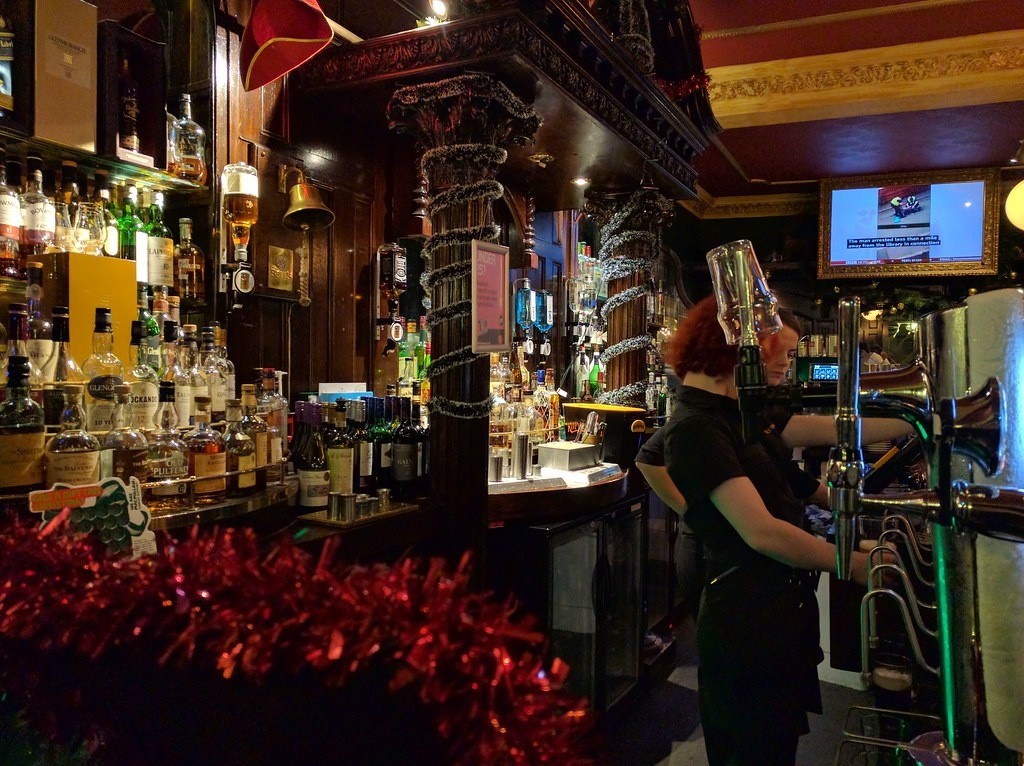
[492,488,652,740]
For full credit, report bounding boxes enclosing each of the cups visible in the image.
[706,239,783,345]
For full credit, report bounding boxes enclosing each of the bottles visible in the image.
[489,239,677,454]
[0,47,431,512]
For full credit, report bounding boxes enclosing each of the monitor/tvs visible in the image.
[809,361,838,384]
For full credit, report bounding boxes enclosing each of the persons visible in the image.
[742,300,922,765]
[890,193,921,218]
[631,290,901,765]
